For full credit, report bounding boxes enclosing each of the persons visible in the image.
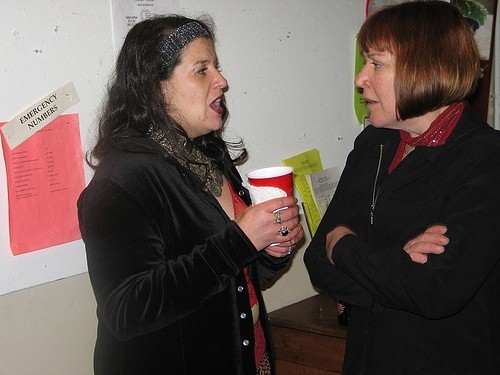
[76,13,304,375]
[304,0,500,374]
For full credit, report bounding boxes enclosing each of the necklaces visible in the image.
[404,143,411,154]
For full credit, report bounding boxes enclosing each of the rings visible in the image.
[273,211,282,224]
[288,246,293,254]
[279,225,290,236]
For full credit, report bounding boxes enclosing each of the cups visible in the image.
[246,167,294,245]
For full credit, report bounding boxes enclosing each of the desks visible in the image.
[265,292,346,375]
